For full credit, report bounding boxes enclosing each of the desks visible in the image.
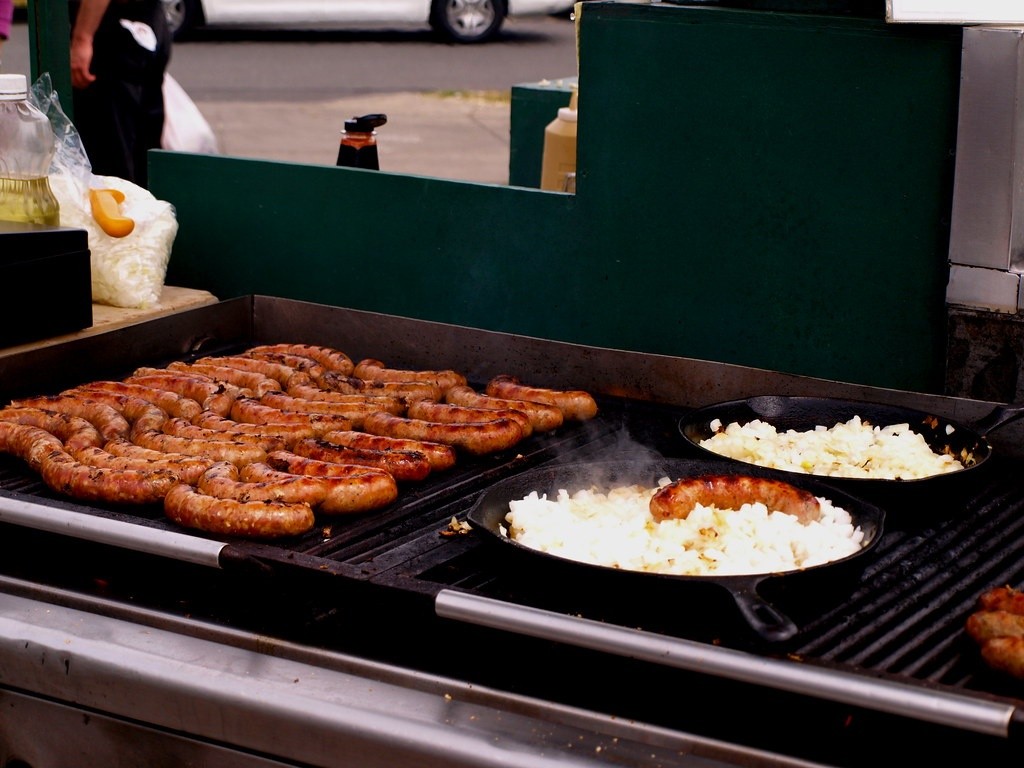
[0,286,219,359]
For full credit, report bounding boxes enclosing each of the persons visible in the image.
[63,0,172,185]
[0,0,13,48]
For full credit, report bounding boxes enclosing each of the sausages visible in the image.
[646,475,821,525]
[0,344,596,536]
[967,585,1024,679]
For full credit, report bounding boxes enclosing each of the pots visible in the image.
[464,456,887,641]
[678,394,1024,495]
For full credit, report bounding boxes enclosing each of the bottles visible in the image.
[337,113,389,172]
[541,89,576,191]
[0,73,61,228]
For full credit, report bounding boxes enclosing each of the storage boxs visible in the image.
[0,220,93,349]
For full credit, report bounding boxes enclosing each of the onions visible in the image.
[699,415,966,479]
[498,476,864,577]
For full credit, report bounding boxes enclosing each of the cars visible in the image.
[161,1,577,48]
